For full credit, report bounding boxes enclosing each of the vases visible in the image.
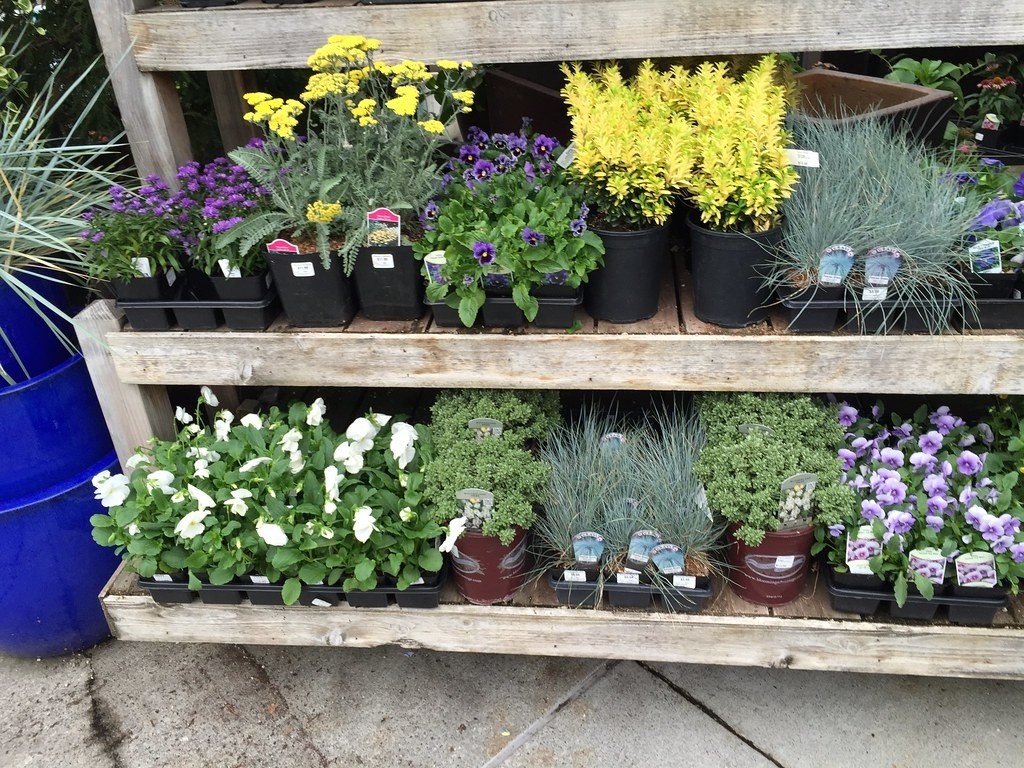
[770,66,953,157]
[136,519,1010,629]
[486,61,576,150]
[111,208,1024,333]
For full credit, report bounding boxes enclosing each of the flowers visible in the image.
[78,33,1024,293]
[90,385,1024,566]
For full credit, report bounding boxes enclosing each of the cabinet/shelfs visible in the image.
[70,0,1024,683]
